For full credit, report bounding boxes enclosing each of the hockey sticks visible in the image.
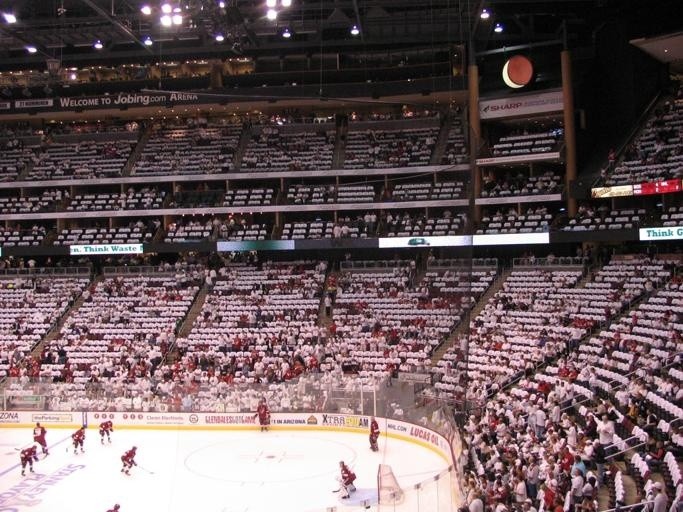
[14,441,35,451]
[332,464,355,493]
[139,466,155,475]
[33,453,47,462]
[66,444,73,451]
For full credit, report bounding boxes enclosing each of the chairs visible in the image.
[0,271,466,394]
[467,262,683,512]
[0,182,683,248]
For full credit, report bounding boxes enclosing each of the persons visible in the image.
[3,101,682,512]
